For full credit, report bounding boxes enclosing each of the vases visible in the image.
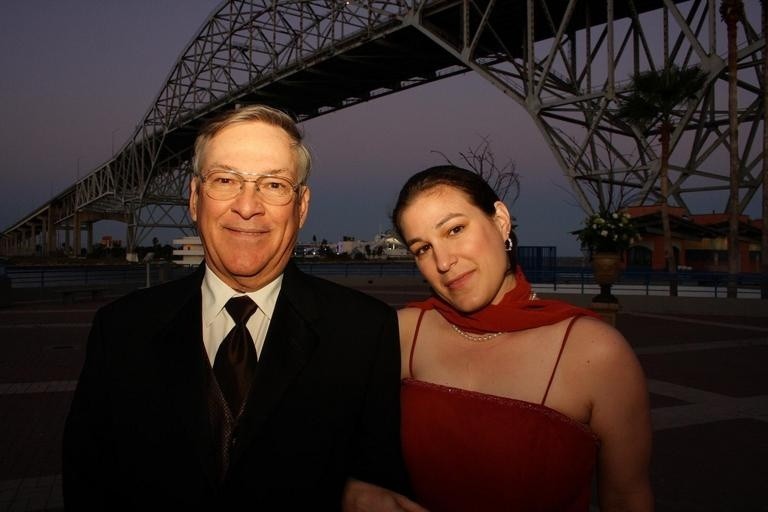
[591,250,621,309]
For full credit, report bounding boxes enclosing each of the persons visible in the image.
[335,165,656,512]
[59,103,401,512]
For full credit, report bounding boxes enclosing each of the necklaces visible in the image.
[452,324,502,341]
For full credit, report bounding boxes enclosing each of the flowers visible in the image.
[569,204,643,263]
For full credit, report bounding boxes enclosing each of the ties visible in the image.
[211,296,257,417]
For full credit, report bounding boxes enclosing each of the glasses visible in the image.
[194,170,302,206]
